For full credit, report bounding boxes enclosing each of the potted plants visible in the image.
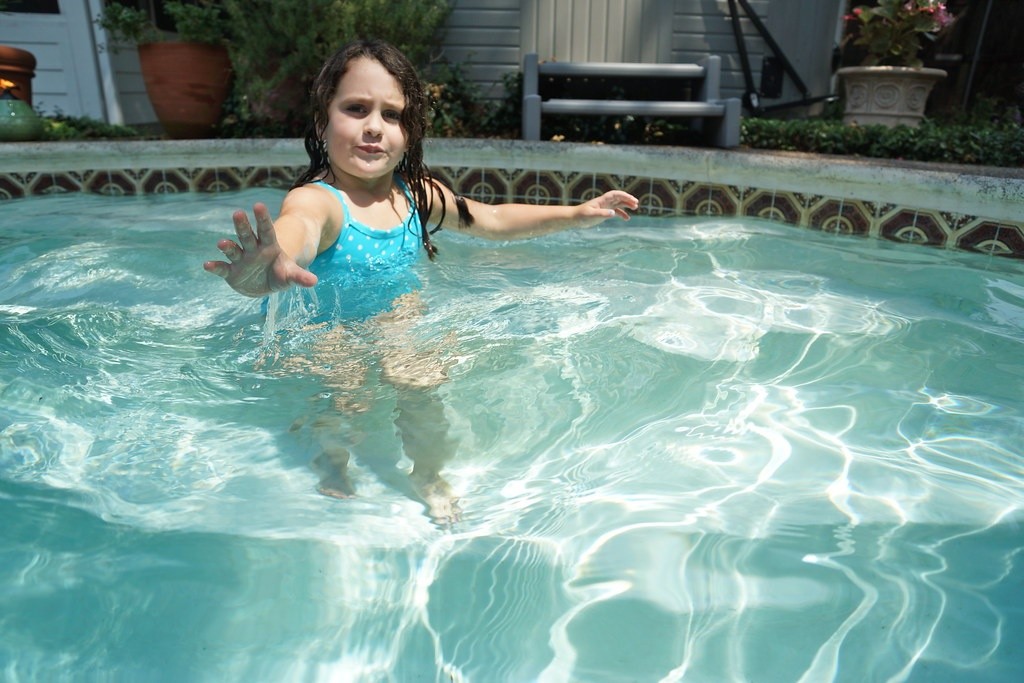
[99,0,231,137]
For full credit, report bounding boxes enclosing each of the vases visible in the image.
[0,42,37,116]
[837,69,945,130]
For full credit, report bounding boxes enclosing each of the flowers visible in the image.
[840,0,965,67]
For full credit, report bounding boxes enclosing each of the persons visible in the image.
[203,36,639,528]
